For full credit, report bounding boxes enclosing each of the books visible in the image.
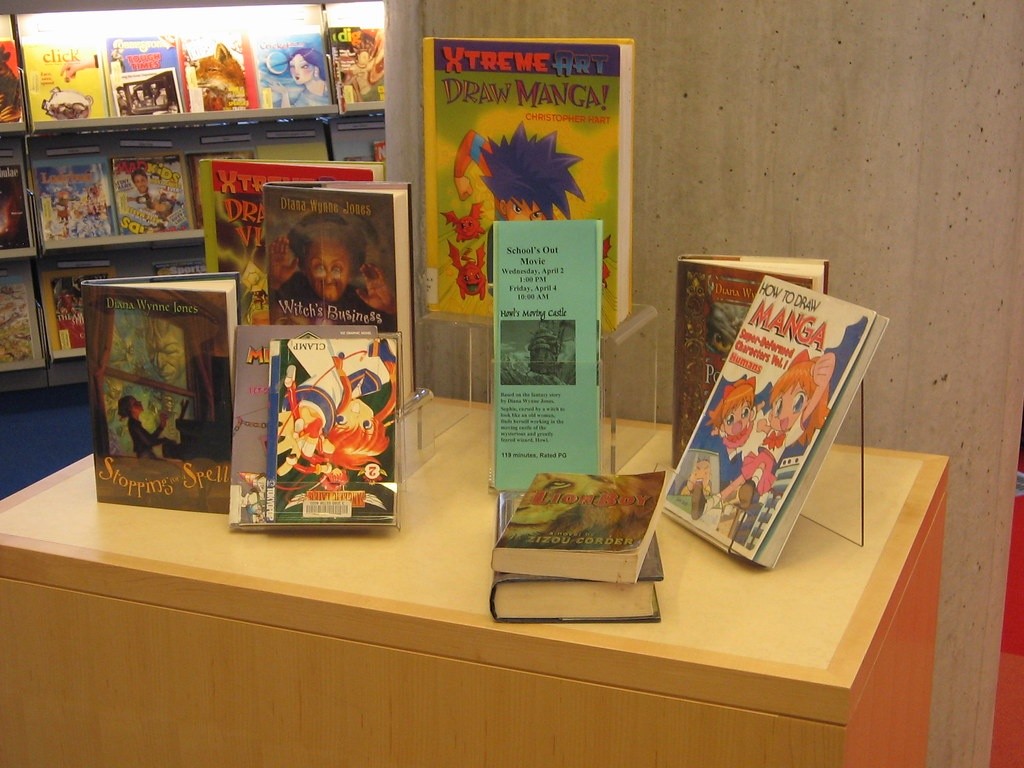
[0,0,890,621]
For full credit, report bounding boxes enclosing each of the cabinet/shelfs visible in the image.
[0,101,385,259]
[0,396,949,768]
